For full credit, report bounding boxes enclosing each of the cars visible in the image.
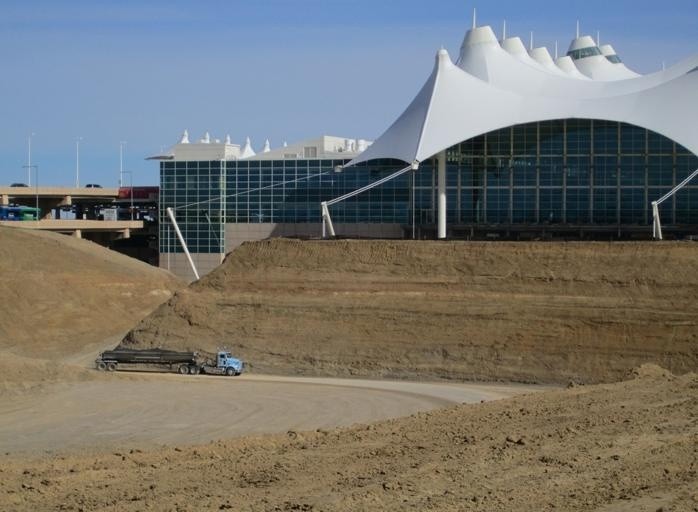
[9,182,28,187]
[85,183,103,188]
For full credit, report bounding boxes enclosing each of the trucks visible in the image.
[0,205,20,220]
[19,207,41,220]
[94,351,241,376]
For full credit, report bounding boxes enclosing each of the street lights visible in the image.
[120,139,128,188]
[24,132,35,187]
[22,165,40,220]
[73,137,84,186]
[119,171,134,220]
